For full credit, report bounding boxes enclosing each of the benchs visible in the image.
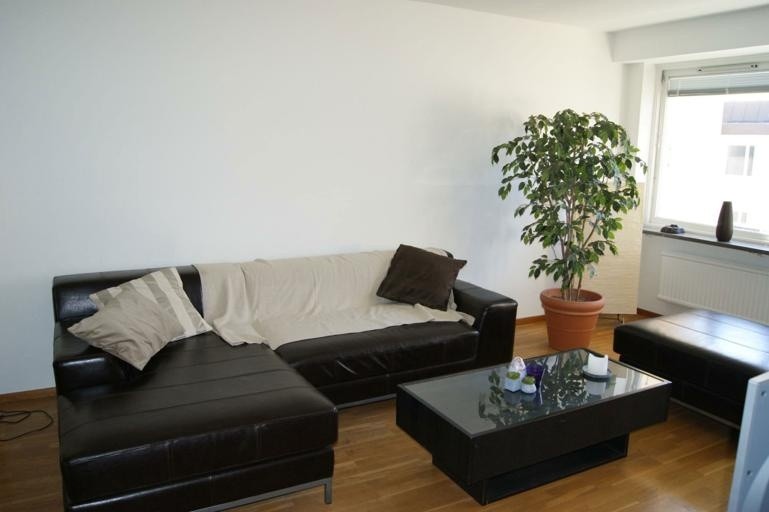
[612,309,769,427]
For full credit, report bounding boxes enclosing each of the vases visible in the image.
[716,199,731,242]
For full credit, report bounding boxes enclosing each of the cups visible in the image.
[588,352,608,374]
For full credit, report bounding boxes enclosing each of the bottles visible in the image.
[504,359,546,393]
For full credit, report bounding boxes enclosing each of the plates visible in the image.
[582,365,613,379]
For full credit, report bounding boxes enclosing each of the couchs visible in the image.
[51,250,519,511]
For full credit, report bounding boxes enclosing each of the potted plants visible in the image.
[493,109,646,353]
[504,371,519,390]
[521,377,537,393]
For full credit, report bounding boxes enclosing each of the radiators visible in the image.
[658,252,769,326]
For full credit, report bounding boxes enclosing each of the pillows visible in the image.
[90,268,211,341]
[66,283,186,370]
[377,244,467,311]
